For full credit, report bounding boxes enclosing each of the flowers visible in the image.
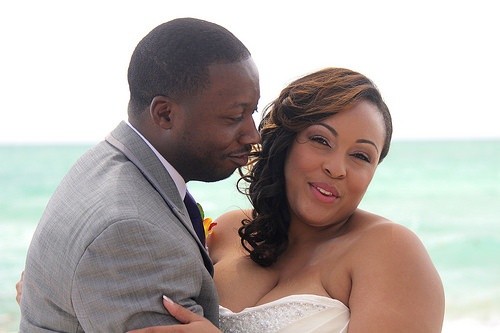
[195,202,217,242]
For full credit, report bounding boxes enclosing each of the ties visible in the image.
[184,193,206,248]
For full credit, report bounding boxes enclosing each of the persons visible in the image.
[17,18,262,333]
[16,68,445,333]
